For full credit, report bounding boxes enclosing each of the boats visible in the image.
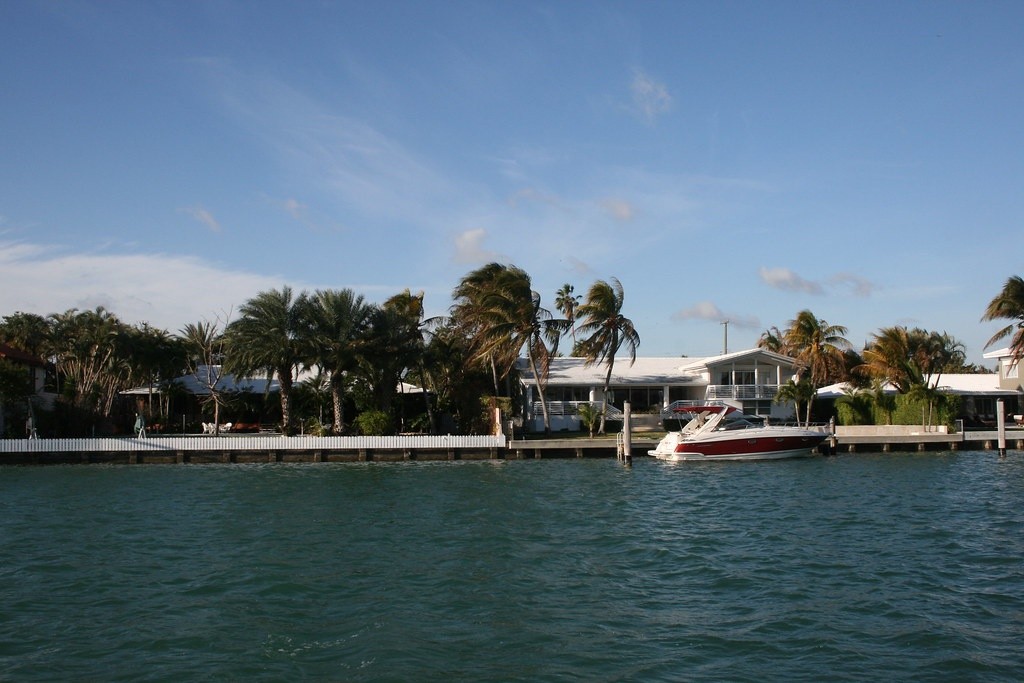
[646,405,830,461]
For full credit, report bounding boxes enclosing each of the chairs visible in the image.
[201,422,232,434]
